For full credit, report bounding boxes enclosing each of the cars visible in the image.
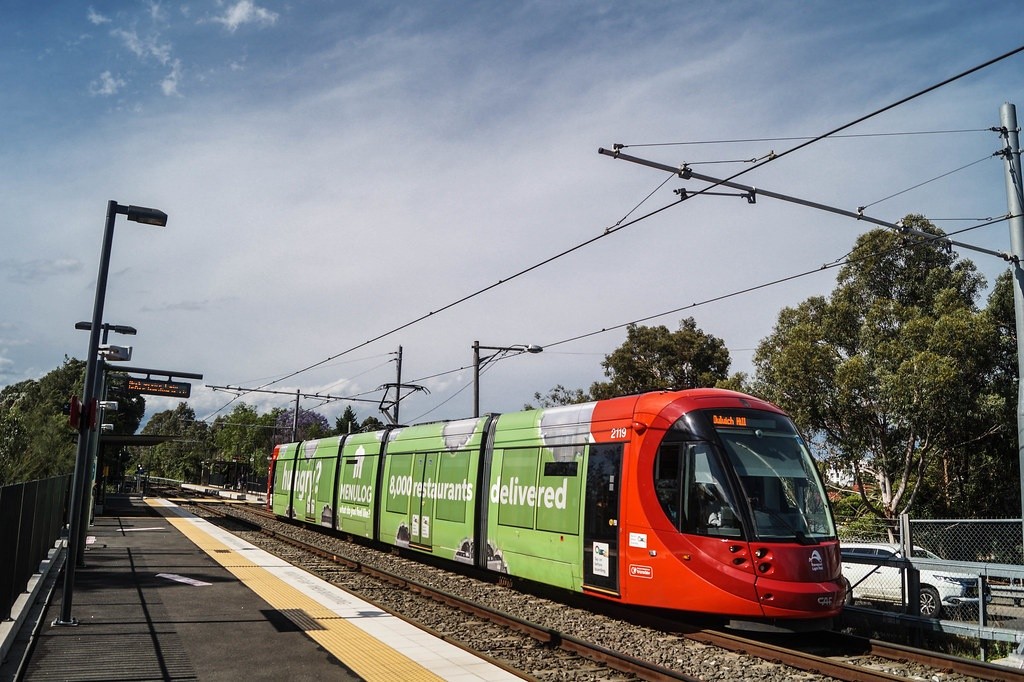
[834,543,993,617]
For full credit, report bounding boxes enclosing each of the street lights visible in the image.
[57,198,168,622]
[72,319,138,566]
[472,341,543,417]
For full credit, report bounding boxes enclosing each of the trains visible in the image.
[265,387,852,621]
